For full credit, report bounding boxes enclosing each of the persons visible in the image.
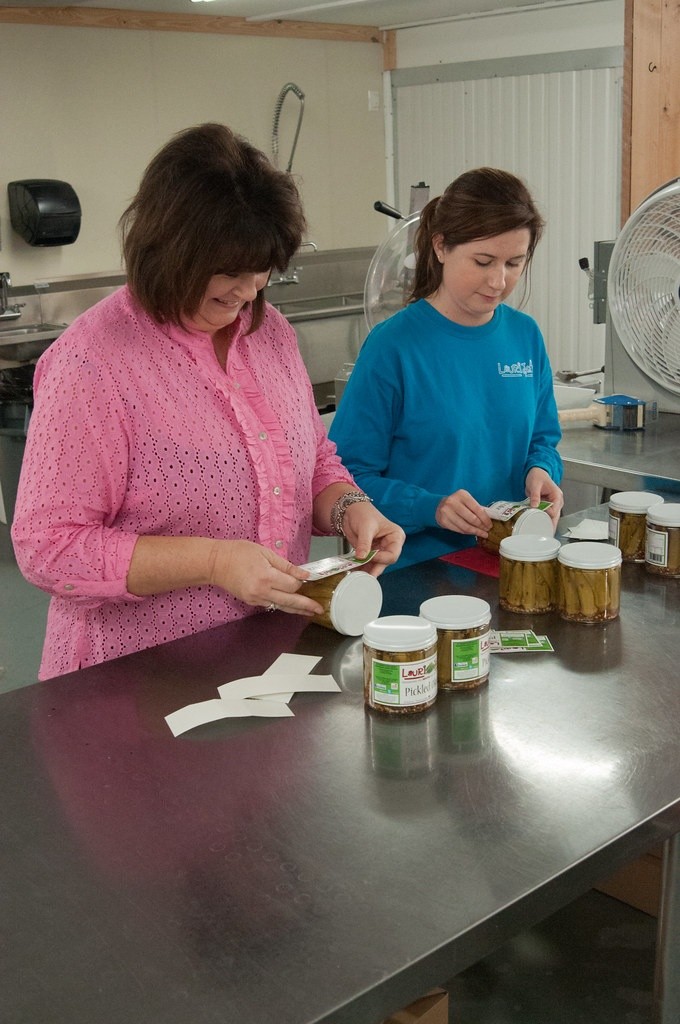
[328,168,564,574]
[10,123,405,682]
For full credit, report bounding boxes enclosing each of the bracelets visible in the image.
[330,491,371,535]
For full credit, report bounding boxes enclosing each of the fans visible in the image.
[605,178,679,396]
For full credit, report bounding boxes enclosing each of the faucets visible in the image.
[0,272,12,314]
[301,241,317,252]
[279,278,297,284]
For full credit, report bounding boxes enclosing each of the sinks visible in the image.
[273,291,403,316]
[0,327,56,337]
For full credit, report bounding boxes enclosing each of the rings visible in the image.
[265,602,275,613]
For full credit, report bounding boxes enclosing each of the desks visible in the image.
[555,410,680,515]
[0,501,680,1024]
